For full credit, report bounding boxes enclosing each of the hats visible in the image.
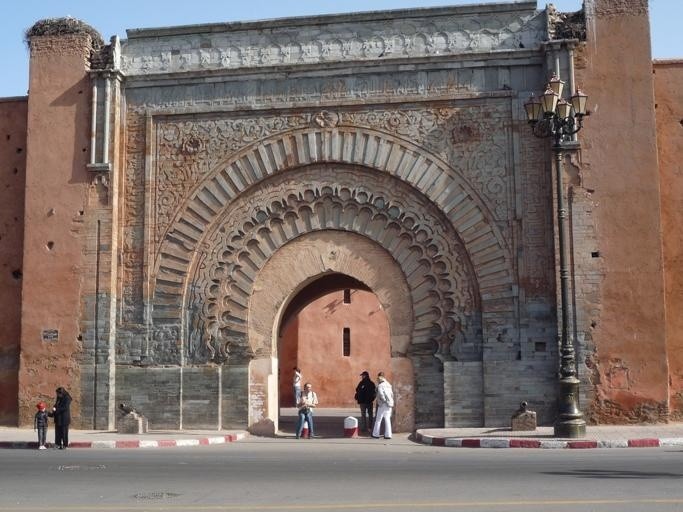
[36,402,45,408]
[359,371,368,375]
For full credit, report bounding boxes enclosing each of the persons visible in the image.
[368,371,395,439]
[49,385,73,451]
[33,401,53,450]
[291,365,302,404]
[352,369,376,435]
[296,382,318,439]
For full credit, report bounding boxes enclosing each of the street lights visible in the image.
[522,72,596,438]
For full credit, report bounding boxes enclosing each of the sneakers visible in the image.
[38,445,47,450]
